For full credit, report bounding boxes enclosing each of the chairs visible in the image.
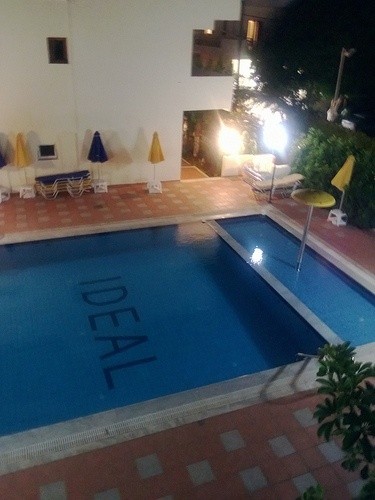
[243,154,305,200]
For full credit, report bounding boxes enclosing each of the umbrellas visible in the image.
[87,130,109,163]
[0,138,8,169]
[331,155,357,194]
[147,131,166,164]
[14,132,35,170]
